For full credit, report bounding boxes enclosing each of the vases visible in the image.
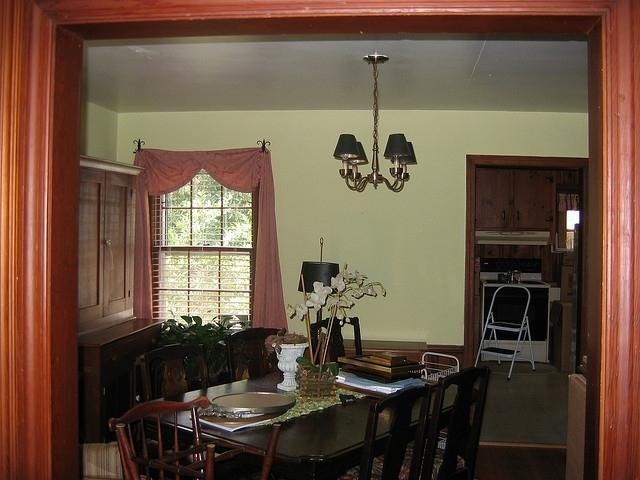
[295,361,339,398]
[272,344,309,392]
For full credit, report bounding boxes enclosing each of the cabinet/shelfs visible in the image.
[552,267,580,373]
[77,156,168,442]
[475,168,583,281]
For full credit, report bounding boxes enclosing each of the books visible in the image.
[338,352,426,382]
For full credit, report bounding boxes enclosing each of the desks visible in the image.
[135,353,488,480]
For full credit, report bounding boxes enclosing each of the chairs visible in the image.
[131,425,282,480]
[308,316,362,363]
[421,365,492,480]
[145,342,209,393]
[360,383,432,480]
[226,328,287,383]
[473,285,536,381]
[109,396,209,480]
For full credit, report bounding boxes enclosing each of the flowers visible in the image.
[287,259,386,377]
[264,328,308,355]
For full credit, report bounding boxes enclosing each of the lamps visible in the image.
[333,55,416,192]
[297,237,339,363]
